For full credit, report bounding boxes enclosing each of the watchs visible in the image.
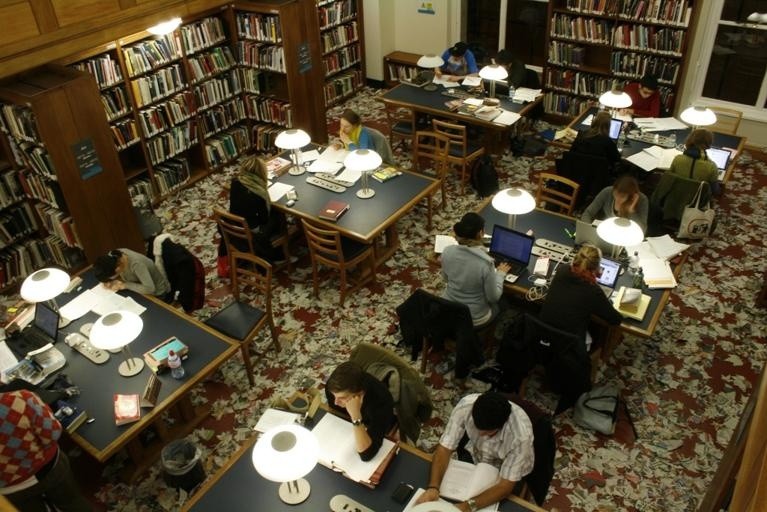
[465,497,479,510]
[350,418,364,427]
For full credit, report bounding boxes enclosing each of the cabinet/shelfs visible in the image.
[231,0,328,152]
[47,0,252,210]
[383,51,435,90]
[0,63,149,278]
[543,0,703,126]
[314,0,367,112]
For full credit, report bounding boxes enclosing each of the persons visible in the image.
[325,360,398,462]
[228,152,299,268]
[413,391,536,512]
[94,245,171,304]
[441,211,511,332]
[581,174,648,235]
[540,243,622,357]
[562,111,622,182]
[491,47,524,90]
[433,41,480,83]
[0,388,83,512]
[339,104,375,154]
[669,128,718,188]
[621,73,660,118]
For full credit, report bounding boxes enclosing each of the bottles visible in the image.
[633,267,644,288]
[168,351,184,378]
[669,132,676,144]
[628,251,640,273]
[509,84,515,101]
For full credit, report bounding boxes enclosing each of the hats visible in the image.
[448,41,468,58]
[494,49,512,67]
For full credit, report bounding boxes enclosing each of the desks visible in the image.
[426,193,688,339]
[552,106,749,186]
[374,71,544,174]
[265,142,442,291]
[179,390,553,512]
[0,263,241,486]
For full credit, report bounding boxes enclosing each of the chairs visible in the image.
[300,218,377,307]
[350,342,422,445]
[520,313,615,411]
[707,106,743,136]
[648,205,668,240]
[431,118,486,195]
[411,289,496,383]
[211,207,292,294]
[200,252,282,386]
[515,399,555,500]
[382,101,431,170]
[535,173,580,217]
[148,235,202,313]
[651,172,709,260]
[361,125,386,168]
[404,131,450,231]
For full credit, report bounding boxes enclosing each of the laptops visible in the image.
[488,224,536,282]
[705,147,732,181]
[4,302,60,358]
[593,256,622,300]
[483,78,510,99]
[591,114,624,145]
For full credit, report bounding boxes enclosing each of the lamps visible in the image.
[492,187,536,230]
[417,54,445,92]
[479,64,509,98]
[343,149,383,199]
[252,423,319,505]
[89,311,144,377]
[410,501,461,512]
[274,129,311,176]
[680,107,717,134]
[21,268,70,330]
[599,91,632,119]
[596,217,644,278]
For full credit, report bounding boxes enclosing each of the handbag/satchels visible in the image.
[574,385,622,435]
[677,180,718,240]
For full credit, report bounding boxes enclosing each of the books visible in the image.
[73,54,153,211]
[371,166,402,184]
[181,16,251,169]
[141,336,189,375]
[321,21,359,53]
[533,255,550,277]
[550,12,687,57]
[438,458,502,511]
[544,92,599,116]
[0,101,83,334]
[322,41,362,76]
[319,197,349,223]
[657,83,675,112]
[311,411,396,482]
[445,97,520,128]
[318,0,357,31]
[235,12,293,157]
[120,32,201,199]
[265,154,292,180]
[110,393,141,425]
[323,67,363,108]
[608,51,680,85]
[544,66,618,97]
[5,342,66,385]
[554,128,578,144]
[49,396,87,433]
[548,40,585,68]
[565,0,693,28]
[613,284,651,323]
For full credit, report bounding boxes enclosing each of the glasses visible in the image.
[640,86,655,95]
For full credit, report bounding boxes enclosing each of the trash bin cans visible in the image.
[159,439,207,493]
